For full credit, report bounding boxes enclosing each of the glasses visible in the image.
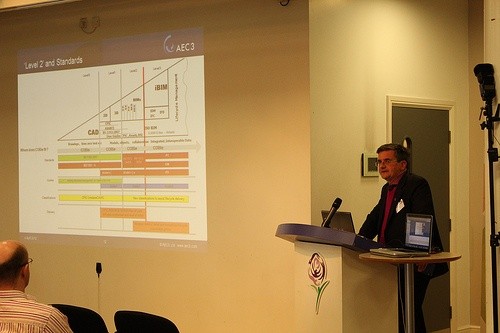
[375,157,397,167]
[27,258,34,265]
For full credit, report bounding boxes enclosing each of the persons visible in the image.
[0,239,74,333]
[356,143,451,333]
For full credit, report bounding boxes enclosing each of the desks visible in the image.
[358,252,462,333]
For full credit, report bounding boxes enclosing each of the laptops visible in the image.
[370,212,434,258]
[321,210,355,233]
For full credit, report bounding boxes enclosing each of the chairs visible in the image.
[114,310,180,333]
[49,304,108,333]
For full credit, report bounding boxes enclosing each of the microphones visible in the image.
[322,198,342,228]
